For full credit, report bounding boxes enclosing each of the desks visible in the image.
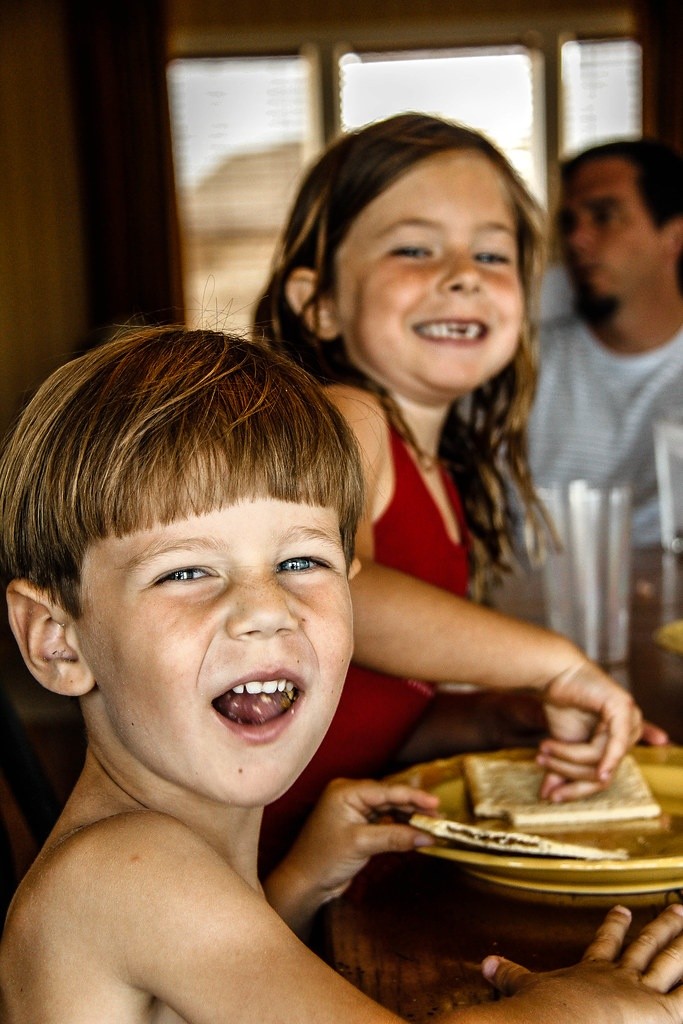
[326,542,683,1023]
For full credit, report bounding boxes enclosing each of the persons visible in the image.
[0,327,683,1024]
[498,135,683,554]
[249,113,565,876]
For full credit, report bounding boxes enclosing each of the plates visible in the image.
[385,748,683,905]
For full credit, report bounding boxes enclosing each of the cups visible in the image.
[655,408,683,552]
[534,481,632,662]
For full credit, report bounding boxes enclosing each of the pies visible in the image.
[463,755,661,823]
[410,816,629,860]
